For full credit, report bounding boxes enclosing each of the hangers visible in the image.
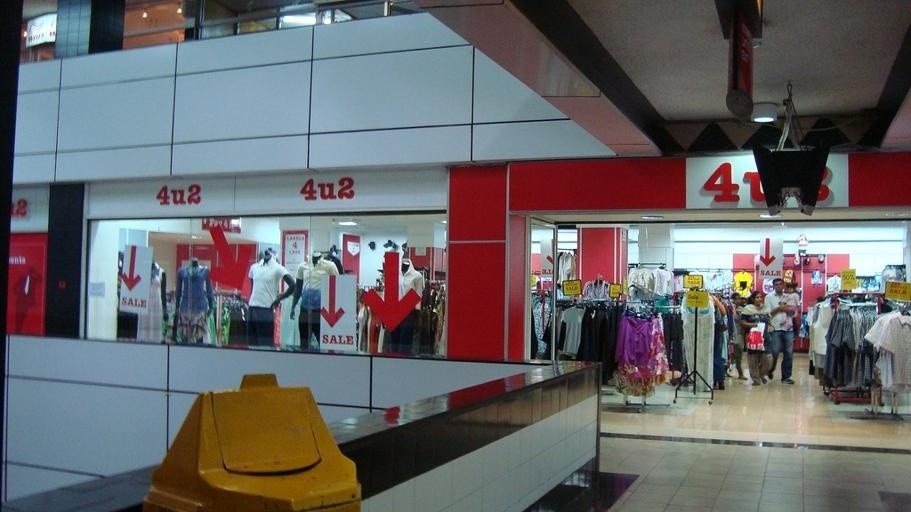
[675,268,732,278]
[557,299,682,322]
[629,262,674,275]
[813,293,911,324]
[557,248,574,258]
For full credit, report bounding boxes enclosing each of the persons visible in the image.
[780,282,801,330]
[740,291,771,386]
[724,292,749,380]
[765,278,799,384]
[247,253,296,345]
[139,261,168,343]
[176,257,215,345]
[290,251,341,348]
[382,258,424,353]
[736,296,748,336]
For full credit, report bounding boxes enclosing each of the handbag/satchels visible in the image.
[746,322,767,352]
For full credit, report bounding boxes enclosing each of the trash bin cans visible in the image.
[145,374,363,512]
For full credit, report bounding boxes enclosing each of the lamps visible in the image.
[750,104,778,123]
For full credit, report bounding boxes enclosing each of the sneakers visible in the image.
[780,319,793,331]
[738,372,794,386]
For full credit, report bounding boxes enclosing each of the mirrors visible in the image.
[526,215,558,364]
